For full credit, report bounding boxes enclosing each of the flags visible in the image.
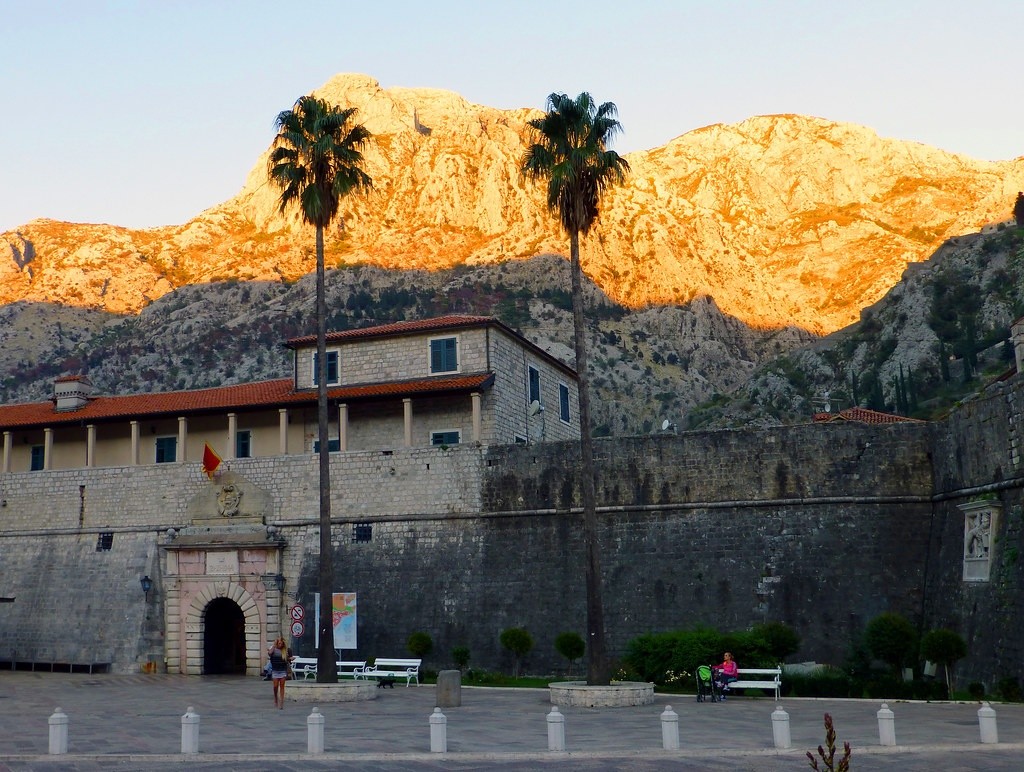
[202,443,220,480]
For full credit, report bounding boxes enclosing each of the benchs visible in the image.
[717,669,782,702]
[289,656,318,680]
[334,661,366,680]
[364,658,422,687]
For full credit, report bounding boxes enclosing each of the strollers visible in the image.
[693,664,722,702]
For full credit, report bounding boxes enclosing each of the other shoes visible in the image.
[723,684,728,690]
[274,698,278,704]
[264,674,271,681]
[279,703,283,710]
[260,670,267,677]
[717,683,723,688]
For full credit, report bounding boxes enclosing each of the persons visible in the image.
[712,652,738,700]
[264,637,296,710]
